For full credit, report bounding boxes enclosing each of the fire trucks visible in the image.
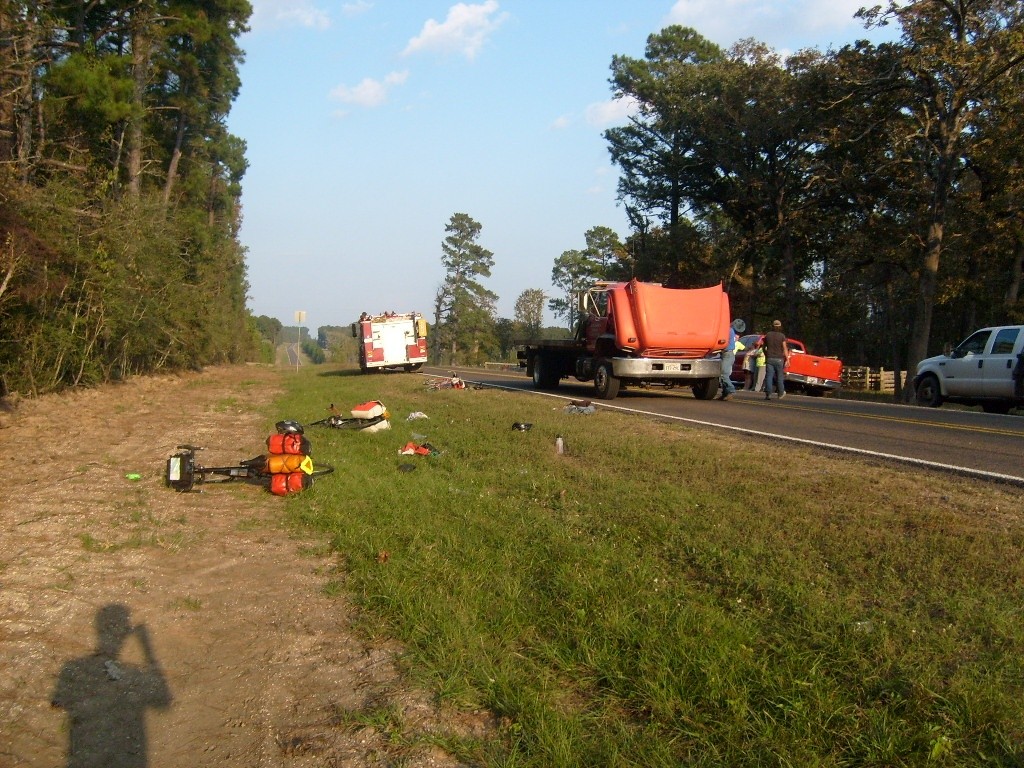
[351,312,427,373]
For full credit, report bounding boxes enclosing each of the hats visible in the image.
[772,320,782,326]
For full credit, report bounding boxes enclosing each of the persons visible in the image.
[762,319,790,399]
[752,338,767,392]
[717,325,737,401]
[741,342,757,390]
[731,332,751,356]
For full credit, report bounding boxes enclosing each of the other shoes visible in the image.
[739,388,750,392]
[764,395,771,400]
[716,396,725,401]
[779,391,786,399]
[724,393,735,401]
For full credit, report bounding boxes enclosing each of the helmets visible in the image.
[276,420,303,434]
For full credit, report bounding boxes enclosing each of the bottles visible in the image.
[556,434,563,454]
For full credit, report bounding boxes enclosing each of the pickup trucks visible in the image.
[912,324,1024,416]
[729,335,843,397]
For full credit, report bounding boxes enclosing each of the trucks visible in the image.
[514,277,731,401]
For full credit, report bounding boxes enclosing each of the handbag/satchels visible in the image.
[167,452,193,487]
[350,400,387,419]
[266,433,315,495]
[362,419,392,433]
